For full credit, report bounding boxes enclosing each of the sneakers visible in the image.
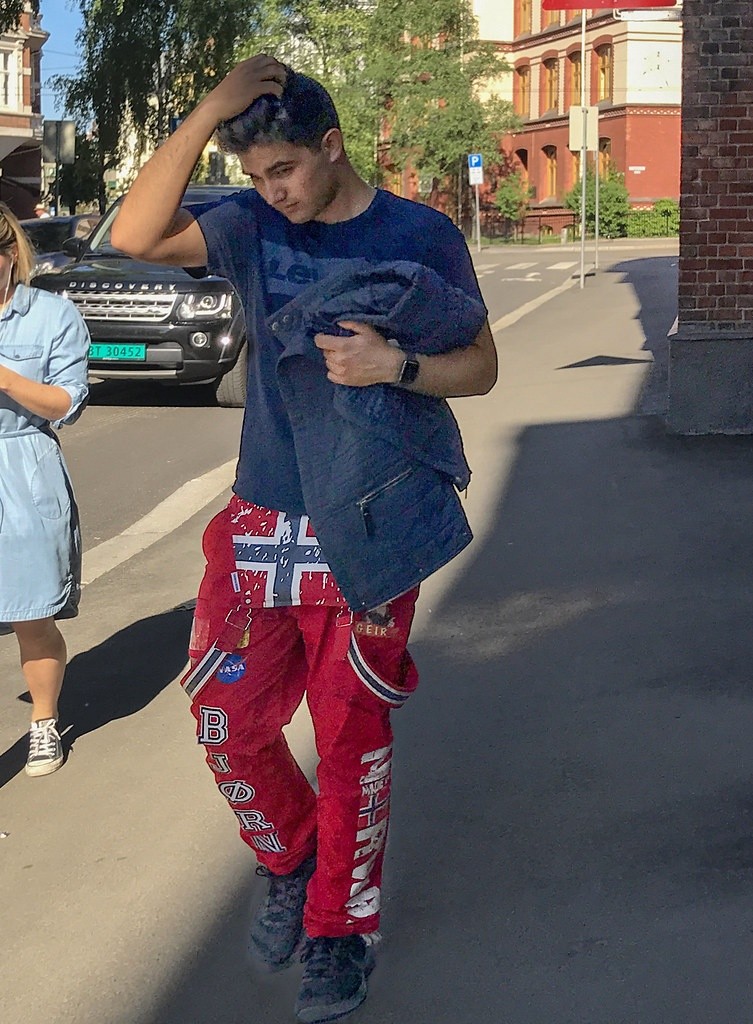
[25,716,74,777]
[295,934,377,1023]
[245,854,318,975]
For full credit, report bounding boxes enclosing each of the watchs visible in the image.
[391,346,420,388]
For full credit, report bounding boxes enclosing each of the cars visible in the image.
[17,214,104,276]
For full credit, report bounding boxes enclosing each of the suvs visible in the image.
[30,186,249,408]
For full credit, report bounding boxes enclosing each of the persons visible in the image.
[34,204,50,219]
[0,197,91,778]
[112,53,497,1024]
